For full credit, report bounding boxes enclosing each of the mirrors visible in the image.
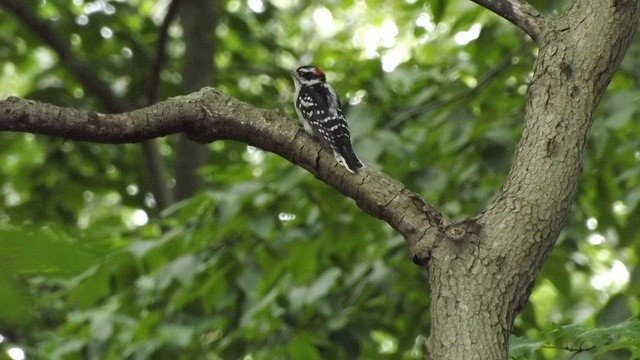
[280,64,366,177]
